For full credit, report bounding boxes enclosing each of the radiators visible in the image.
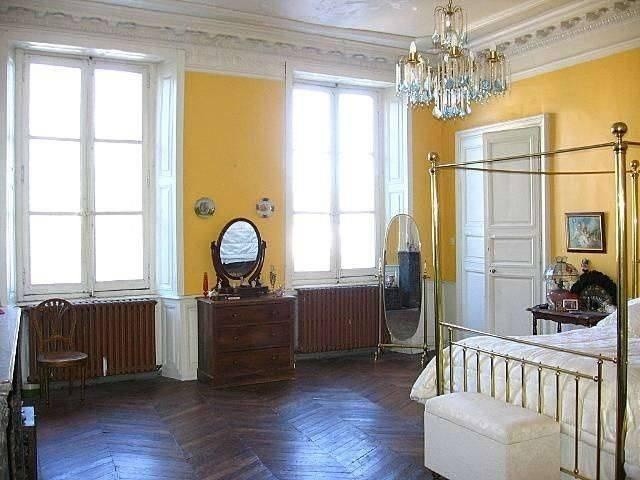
[296,285,379,353]
[25,299,160,386]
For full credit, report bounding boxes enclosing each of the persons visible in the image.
[573,223,598,247]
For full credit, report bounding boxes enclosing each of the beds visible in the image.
[410,295,640,479]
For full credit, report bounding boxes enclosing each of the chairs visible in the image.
[32,300,88,405]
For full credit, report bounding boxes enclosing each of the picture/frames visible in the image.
[565,212,606,253]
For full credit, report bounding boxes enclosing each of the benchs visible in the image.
[422,393,562,479]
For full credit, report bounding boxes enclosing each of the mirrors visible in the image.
[374,210,430,367]
[210,218,266,290]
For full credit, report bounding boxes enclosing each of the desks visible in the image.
[528,306,610,335]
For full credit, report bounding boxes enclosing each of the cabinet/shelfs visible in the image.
[196,296,294,387]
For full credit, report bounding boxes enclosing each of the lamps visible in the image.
[393,0,510,121]
[544,254,579,306]
